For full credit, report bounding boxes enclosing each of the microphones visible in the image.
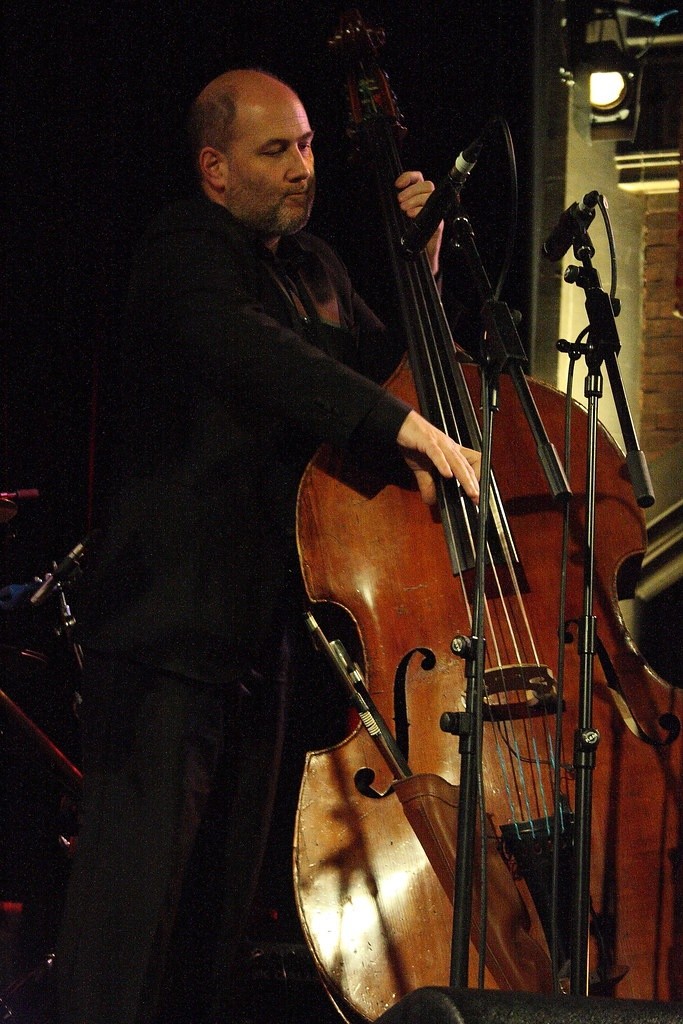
[393,117,506,259]
[29,543,84,608]
[540,190,607,262]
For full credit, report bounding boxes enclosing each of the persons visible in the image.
[54,69,481,1024]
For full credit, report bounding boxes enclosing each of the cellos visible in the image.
[286,65,679,1022]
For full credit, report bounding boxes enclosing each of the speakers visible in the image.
[370,986,683,1024]
